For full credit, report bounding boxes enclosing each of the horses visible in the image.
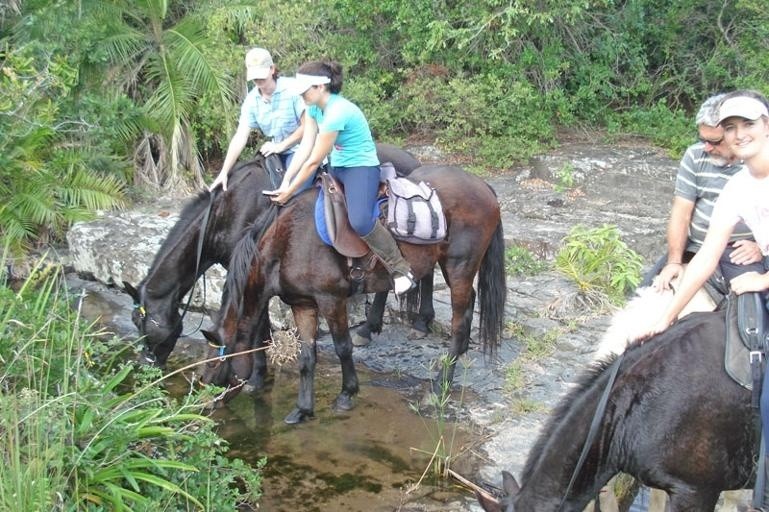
[122,141,437,393]
[199,162,507,425]
[475,303,768,512]
[582,278,742,512]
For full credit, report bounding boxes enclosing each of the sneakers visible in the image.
[393,272,414,295]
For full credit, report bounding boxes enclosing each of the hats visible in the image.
[244,48,274,81]
[287,72,332,95]
[715,96,768,128]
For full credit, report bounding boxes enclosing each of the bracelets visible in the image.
[666,262,682,265]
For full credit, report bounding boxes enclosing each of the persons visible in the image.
[637,93,766,295]
[652,89,769,512]
[270,60,414,294]
[208,48,318,197]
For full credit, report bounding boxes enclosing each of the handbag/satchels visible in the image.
[386,177,447,245]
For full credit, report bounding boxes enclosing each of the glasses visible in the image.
[698,137,724,145]
